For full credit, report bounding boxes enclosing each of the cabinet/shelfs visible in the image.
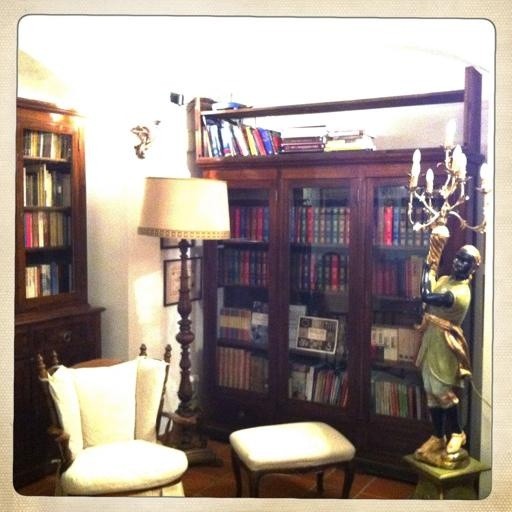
[16,106,103,488]
[192,64,481,483]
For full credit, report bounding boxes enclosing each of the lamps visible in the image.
[136,178,232,472]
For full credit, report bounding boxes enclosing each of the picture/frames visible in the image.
[161,237,195,250]
[163,256,202,308]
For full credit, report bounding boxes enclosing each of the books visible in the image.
[228,206,270,241]
[200,109,383,155]
[216,346,268,392]
[289,360,348,407]
[375,206,432,247]
[290,252,349,295]
[370,324,421,362]
[288,305,346,355]
[217,249,267,287]
[371,255,424,299]
[220,302,270,344]
[370,375,424,418]
[289,206,350,245]
[22,128,75,299]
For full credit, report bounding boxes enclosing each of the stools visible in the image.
[229,419,356,498]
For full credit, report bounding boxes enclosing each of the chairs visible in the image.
[35,345,187,498]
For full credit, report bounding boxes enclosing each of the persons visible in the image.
[402,224,481,456]
